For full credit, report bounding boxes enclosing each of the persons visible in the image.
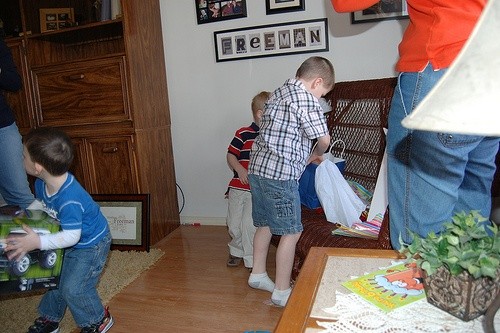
[226,56,335,307]
[331,0,500,252]
[2,127,114,333]
[0,40,43,210]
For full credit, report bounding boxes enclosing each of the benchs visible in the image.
[270,76,500,281]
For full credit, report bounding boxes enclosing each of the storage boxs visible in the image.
[0,216,65,301]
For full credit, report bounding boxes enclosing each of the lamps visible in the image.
[404,0,499,136]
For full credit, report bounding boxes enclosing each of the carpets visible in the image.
[0,249,166,333]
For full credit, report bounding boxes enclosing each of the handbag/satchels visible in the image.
[298,140,345,209]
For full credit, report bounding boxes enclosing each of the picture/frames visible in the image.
[195,0,249,25]
[89,194,150,254]
[213,18,330,62]
[351,0,411,24]
[265,0,305,15]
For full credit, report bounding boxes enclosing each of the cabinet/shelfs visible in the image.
[1,0,182,246]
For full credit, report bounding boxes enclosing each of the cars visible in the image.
[0,226,58,279]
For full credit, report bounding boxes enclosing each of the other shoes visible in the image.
[247,268,252,273]
[227,255,243,266]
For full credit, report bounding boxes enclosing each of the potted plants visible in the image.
[409,211,500,322]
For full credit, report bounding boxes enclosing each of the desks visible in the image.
[274,246,500,333]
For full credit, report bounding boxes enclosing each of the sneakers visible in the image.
[79,308,114,333]
[27,316,60,333]
